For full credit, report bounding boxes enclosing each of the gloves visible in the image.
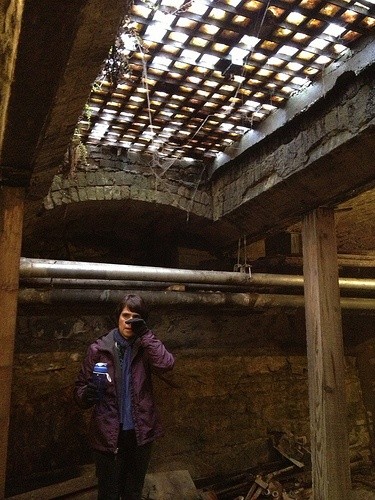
[125,318,149,336]
[83,383,99,404]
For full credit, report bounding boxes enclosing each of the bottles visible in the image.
[91,363,111,402]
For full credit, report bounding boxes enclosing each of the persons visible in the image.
[71,295,176,500]
